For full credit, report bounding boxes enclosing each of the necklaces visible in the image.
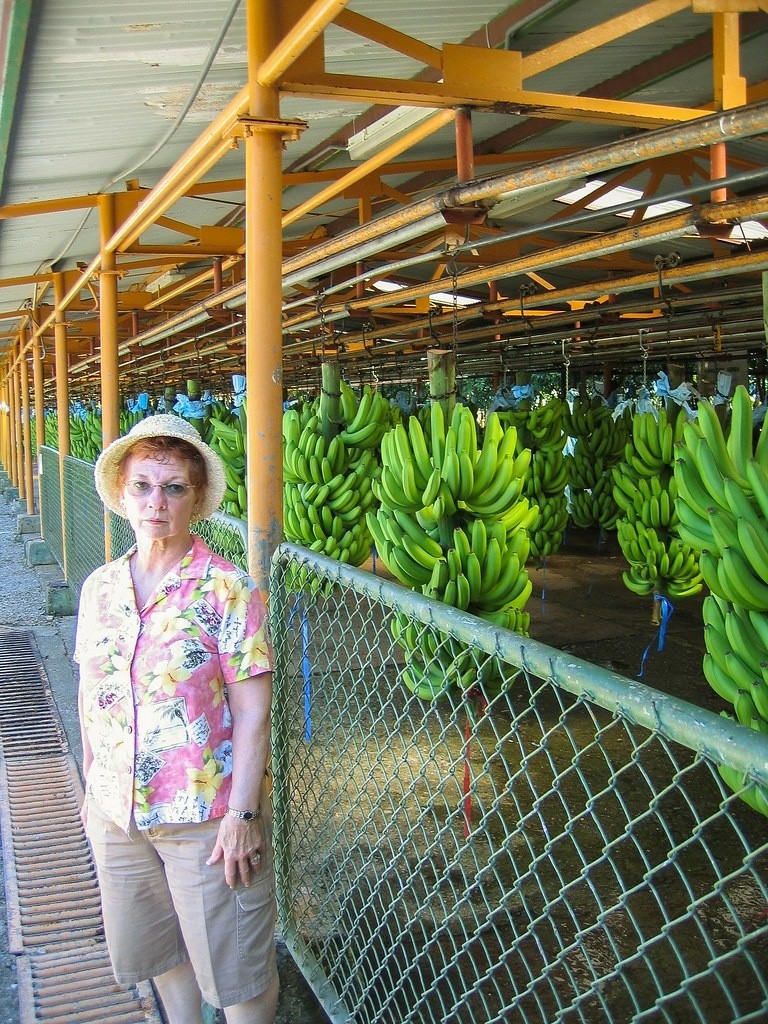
[133,555,145,608]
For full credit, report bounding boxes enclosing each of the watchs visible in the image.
[227,802,261,823]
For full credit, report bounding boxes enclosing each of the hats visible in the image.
[93,414,227,525]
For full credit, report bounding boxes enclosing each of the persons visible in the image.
[72,413,279,1024]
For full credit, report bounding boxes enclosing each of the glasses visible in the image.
[123,479,197,499]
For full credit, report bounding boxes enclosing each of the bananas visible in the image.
[21,385,768,821]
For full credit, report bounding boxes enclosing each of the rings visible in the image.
[251,854,260,862]
